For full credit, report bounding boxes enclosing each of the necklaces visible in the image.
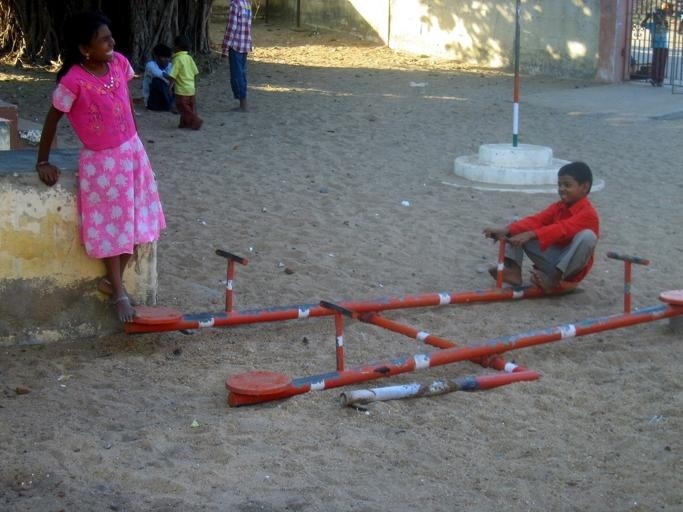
[77,60,115,90]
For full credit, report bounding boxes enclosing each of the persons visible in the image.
[167,34,204,131]
[479,160,601,299]
[141,43,179,115]
[219,1,253,112]
[35,15,167,326]
[640,8,671,88]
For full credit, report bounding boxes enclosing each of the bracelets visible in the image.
[37,159,49,170]
